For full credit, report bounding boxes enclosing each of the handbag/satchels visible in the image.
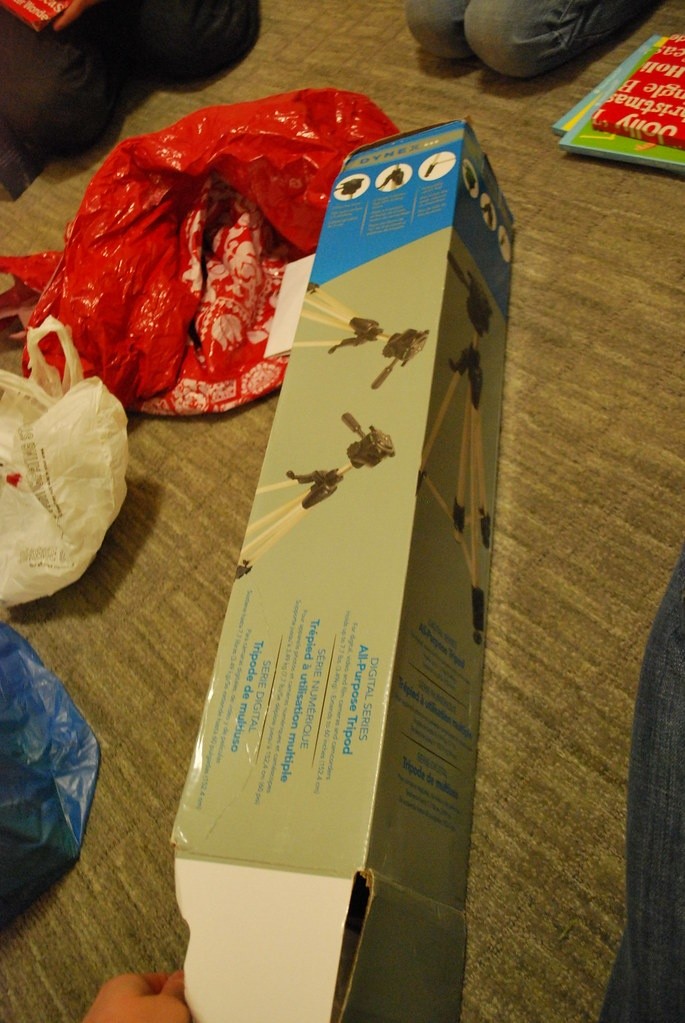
[1,313,129,610]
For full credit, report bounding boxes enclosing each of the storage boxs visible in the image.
[170,119,514,1023]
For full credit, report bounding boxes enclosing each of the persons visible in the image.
[0,0,259,154]
[406,0,658,78]
